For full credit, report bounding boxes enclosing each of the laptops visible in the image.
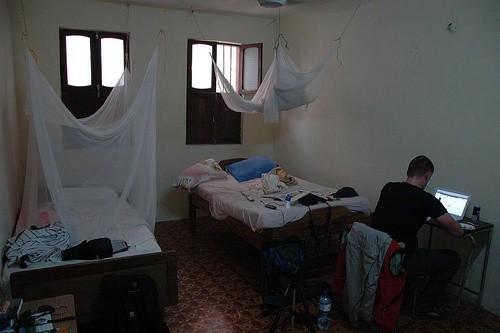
[432,187,471,221]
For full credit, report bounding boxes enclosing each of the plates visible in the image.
[458,223,476,230]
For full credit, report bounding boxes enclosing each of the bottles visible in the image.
[317,290,332,331]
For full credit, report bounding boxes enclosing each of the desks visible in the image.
[19,294,77,333]
[425,217,494,329]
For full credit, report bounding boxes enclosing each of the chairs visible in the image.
[335,223,417,333]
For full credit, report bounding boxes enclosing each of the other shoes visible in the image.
[410,306,448,320]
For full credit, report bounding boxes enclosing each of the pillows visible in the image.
[226,155,275,183]
[172,158,228,192]
[51,188,118,208]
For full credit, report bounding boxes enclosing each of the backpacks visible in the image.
[262,237,309,316]
[101,272,171,333]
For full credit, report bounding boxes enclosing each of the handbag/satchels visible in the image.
[298,193,326,206]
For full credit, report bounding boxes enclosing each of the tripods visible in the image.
[263,262,316,333]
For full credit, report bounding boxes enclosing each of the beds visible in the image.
[189,157,373,296]
[0,185,178,305]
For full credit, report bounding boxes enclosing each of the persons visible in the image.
[371,155,465,315]
